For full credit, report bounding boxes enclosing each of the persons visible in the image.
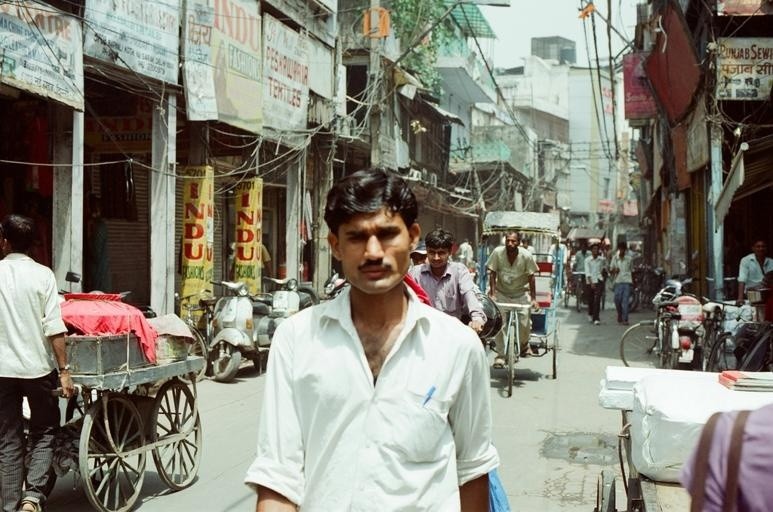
[736,240,773,306]
[679,401,773,512]
[547,242,634,325]
[485,230,540,369]
[408,229,488,335]
[245,169,499,512]
[0,214,75,512]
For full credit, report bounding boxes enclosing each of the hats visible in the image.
[410,241,427,258]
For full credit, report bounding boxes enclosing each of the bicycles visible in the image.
[172,286,217,384]
[614,261,773,372]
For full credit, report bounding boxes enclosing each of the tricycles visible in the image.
[475,209,564,398]
[565,227,610,313]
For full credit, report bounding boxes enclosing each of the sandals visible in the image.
[19,496,42,512]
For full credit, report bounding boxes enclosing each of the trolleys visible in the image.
[11,320,211,511]
[595,408,695,512]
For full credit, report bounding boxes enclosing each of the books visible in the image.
[719,371,773,392]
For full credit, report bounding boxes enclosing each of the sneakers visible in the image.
[588,315,600,325]
[617,316,629,325]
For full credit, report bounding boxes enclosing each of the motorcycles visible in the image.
[207,272,348,382]
